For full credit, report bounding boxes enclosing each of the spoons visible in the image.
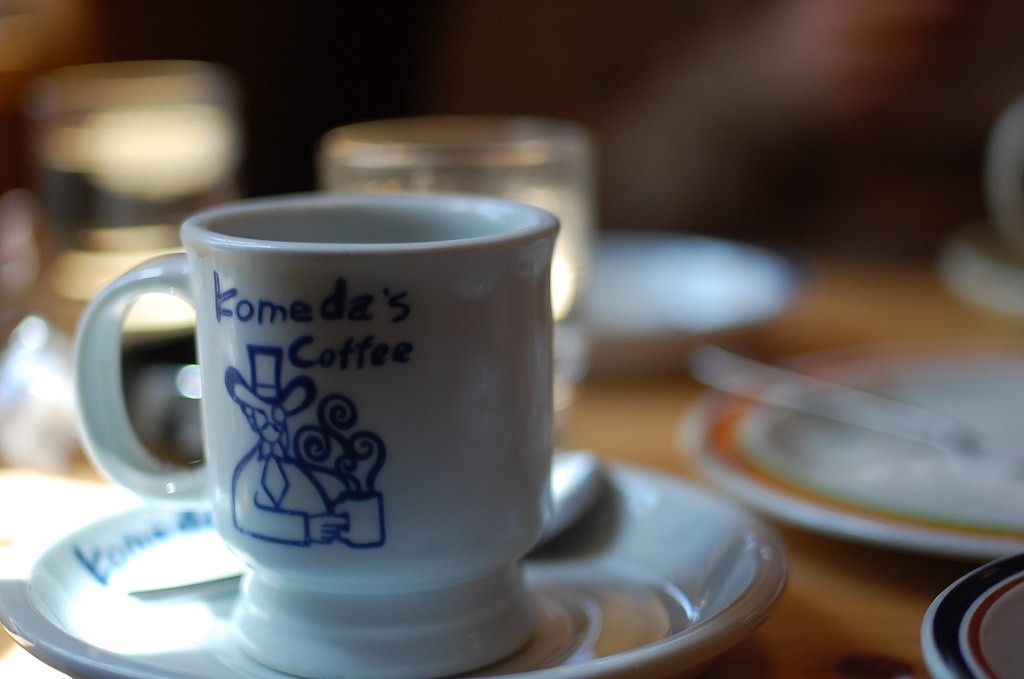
[128,448,600,594]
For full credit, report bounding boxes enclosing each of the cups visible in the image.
[317,115,597,440]
[69,191,556,679]
[22,59,242,335]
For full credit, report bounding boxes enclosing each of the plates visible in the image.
[0,455,789,679]
[577,230,804,375]
[919,550,1024,679]
[673,336,1024,557]
